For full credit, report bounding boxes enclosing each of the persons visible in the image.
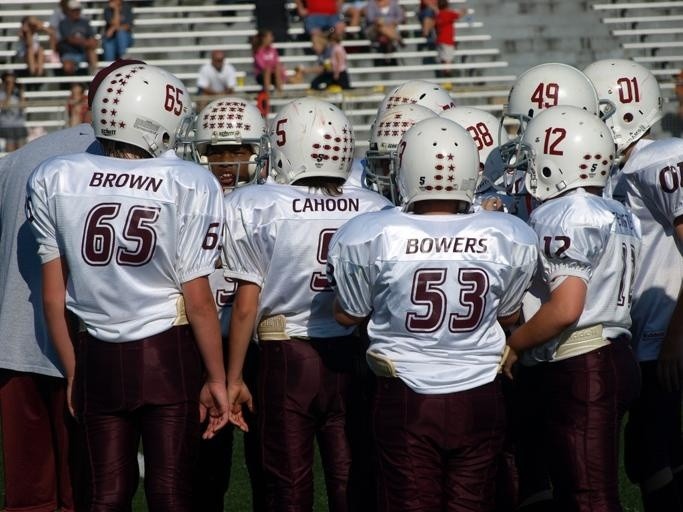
[177,97,271,512]
[12,16,54,77]
[49,1,69,53]
[326,118,539,511]
[0,74,29,153]
[55,0,101,75]
[582,59,682,511]
[0,60,147,512]
[24,65,227,512]
[507,106,642,512]
[249,0,467,94]
[437,105,540,225]
[62,83,92,128]
[195,51,237,115]
[499,61,601,163]
[358,103,441,208]
[226,99,397,511]
[101,0,134,61]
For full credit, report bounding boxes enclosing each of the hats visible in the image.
[88,61,149,112]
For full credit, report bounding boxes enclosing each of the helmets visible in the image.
[439,105,510,191]
[394,117,480,212]
[269,95,355,186]
[504,63,601,122]
[516,92,526,100]
[517,105,616,202]
[194,97,270,187]
[583,58,668,158]
[91,63,191,159]
[362,104,440,196]
